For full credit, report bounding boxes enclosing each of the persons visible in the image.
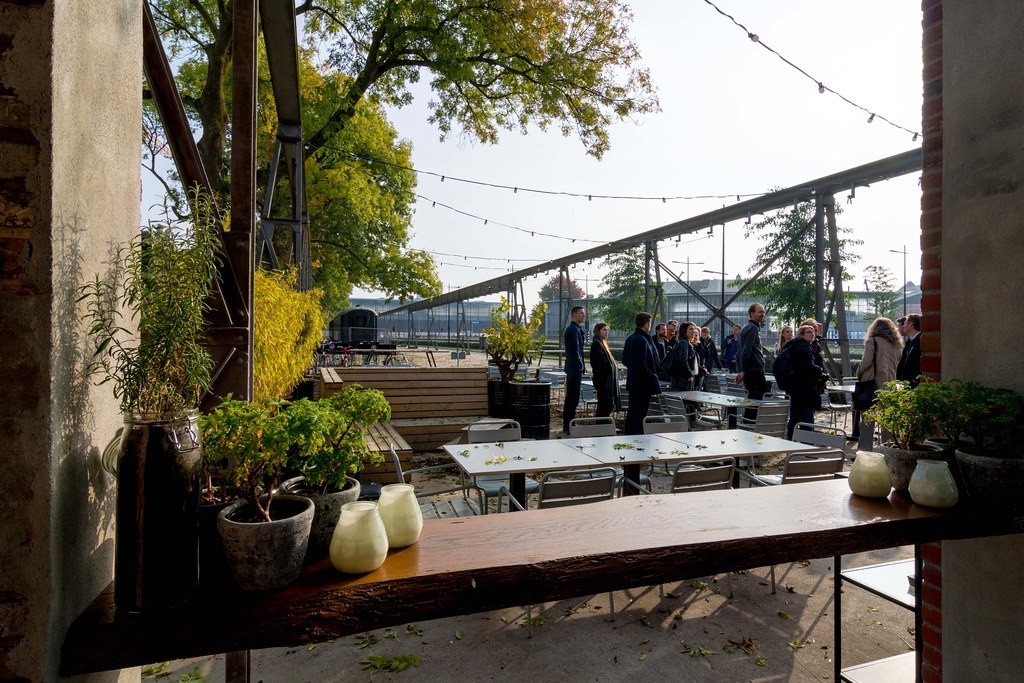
[589,323,622,438]
[850,317,905,462]
[772,318,830,460]
[734,303,771,431]
[622,313,662,435]
[652,320,742,432]
[563,306,585,436]
[895,313,922,390]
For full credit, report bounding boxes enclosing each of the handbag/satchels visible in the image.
[659,344,681,375]
[853,380,878,411]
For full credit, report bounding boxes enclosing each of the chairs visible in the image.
[458,367,851,515]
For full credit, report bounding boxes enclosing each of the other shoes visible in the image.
[563,424,570,434]
[847,435,859,441]
[690,423,704,431]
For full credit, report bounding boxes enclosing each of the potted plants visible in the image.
[860,376,1024,519]
[479,297,553,442]
[274,383,392,551]
[194,388,315,594]
[72,178,227,616]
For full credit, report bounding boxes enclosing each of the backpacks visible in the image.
[772,342,797,389]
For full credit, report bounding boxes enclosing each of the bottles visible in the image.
[328,501,391,574]
[377,483,423,548]
[848,450,891,498]
[907,460,958,508]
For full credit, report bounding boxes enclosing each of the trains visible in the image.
[328,304,379,344]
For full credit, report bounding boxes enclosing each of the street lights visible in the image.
[889,245,908,318]
[672,256,705,323]
[575,275,600,346]
[701,203,731,369]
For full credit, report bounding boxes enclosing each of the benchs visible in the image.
[349,347,450,367]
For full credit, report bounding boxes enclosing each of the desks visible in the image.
[720,370,776,384]
[827,384,856,393]
[545,369,593,381]
[656,428,820,488]
[558,433,716,498]
[581,377,672,393]
[57,480,1023,683]
[663,391,764,429]
[442,436,603,510]
